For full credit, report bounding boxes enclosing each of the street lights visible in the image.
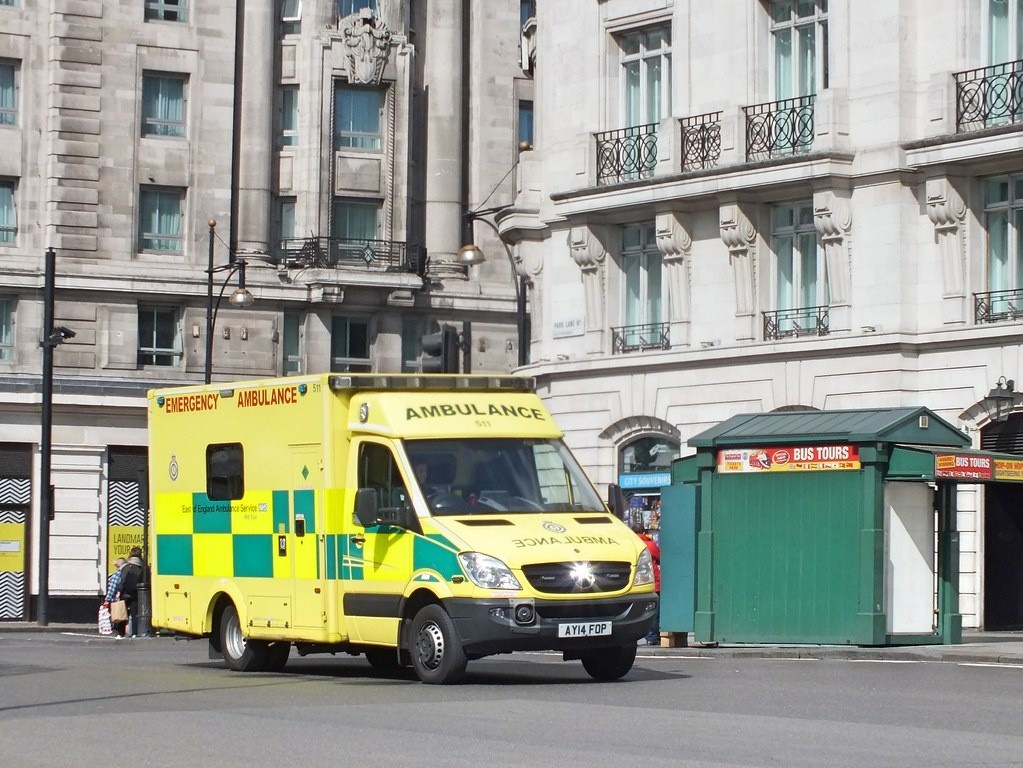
[457,212,528,364]
[204,260,256,384]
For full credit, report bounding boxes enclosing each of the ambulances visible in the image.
[146,372,660,684]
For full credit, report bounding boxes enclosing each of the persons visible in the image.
[630,523,660,646]
[390,454,448,522]
[470,440,540,507]
[99,547,145,640]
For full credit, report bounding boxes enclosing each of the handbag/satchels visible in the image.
[111,598,128,623]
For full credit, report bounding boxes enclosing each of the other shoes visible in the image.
[129,634,137,638]
[115,635,126,640]
[648,639,661,646]
